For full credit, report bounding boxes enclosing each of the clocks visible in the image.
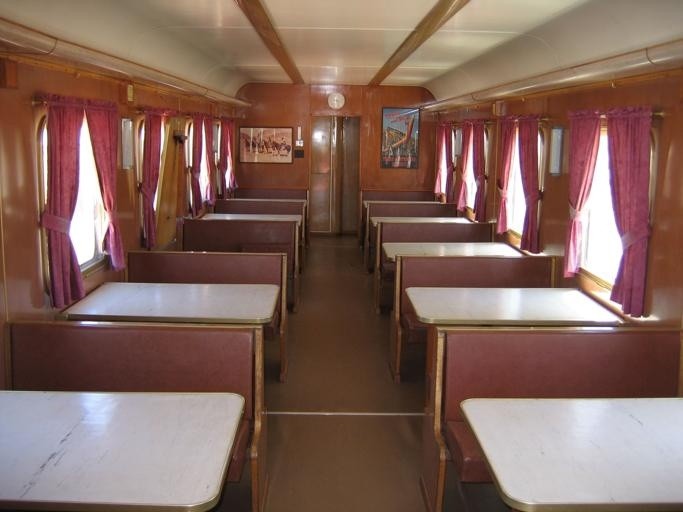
[327,94,345,109]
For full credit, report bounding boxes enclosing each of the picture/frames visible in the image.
[239,125,294,164]
[379,105,422,170]
